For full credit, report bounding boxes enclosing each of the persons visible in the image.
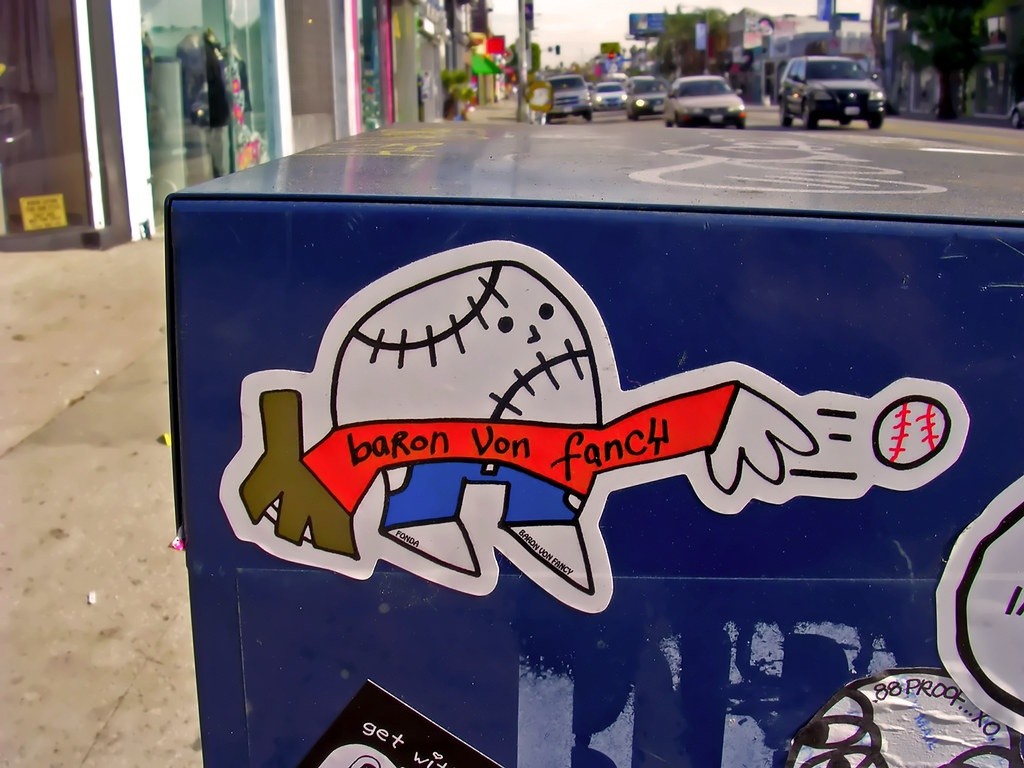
[175,27,253,183]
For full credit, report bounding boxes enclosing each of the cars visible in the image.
[580,71,628,112]
[625,73,668,122]
[778,55,885,134]
[543,73,594,128]
[666,75,747,131]
